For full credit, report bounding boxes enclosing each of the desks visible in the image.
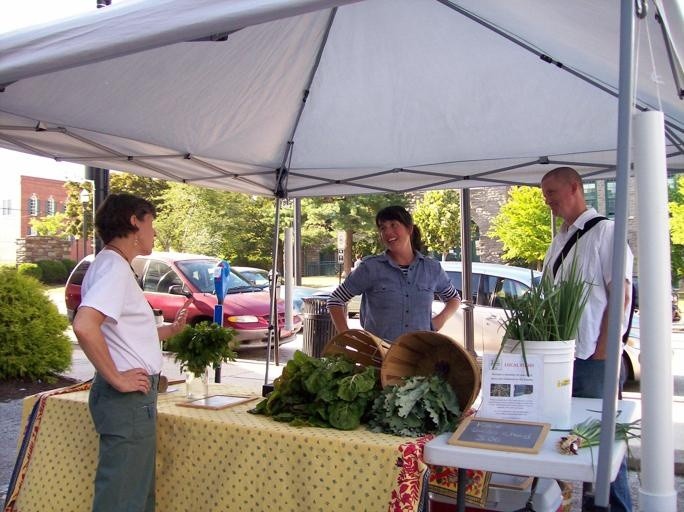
[424,397,637,512]
[4,378,491,512]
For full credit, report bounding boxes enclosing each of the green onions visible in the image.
[557,417,644,485]
[491,230,597,375]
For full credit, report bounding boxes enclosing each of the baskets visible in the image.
[320,328,391,396]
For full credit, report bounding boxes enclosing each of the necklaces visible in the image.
[106,244,145,291]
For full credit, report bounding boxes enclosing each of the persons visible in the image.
[538,167,634,511]
[70,191,189,511]
[326,204,462,370]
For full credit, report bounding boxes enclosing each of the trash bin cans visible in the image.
[301,297,348,361]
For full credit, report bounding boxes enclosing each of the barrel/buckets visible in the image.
[503,339,575,427]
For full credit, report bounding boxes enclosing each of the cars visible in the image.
[431,261,641,382]
[64,251,362,350]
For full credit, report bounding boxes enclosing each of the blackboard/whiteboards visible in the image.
[447,416,551,454]
